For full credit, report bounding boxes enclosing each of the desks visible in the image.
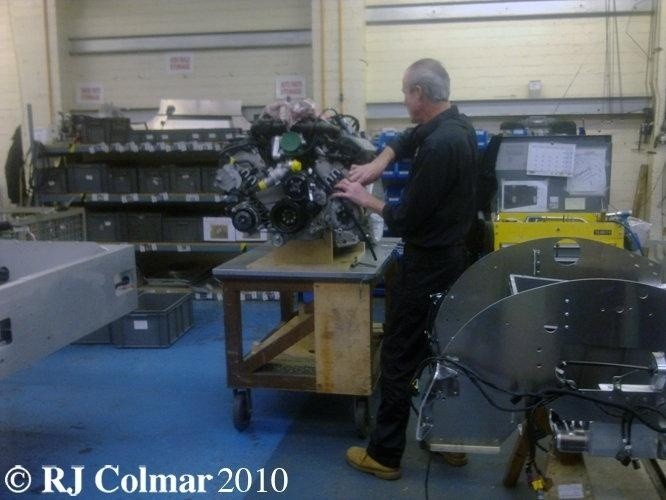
[211,235,404,441]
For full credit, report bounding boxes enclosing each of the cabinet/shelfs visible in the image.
[31,142,271,302]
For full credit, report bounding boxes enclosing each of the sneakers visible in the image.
[437,451,468,467]
[345,445,402,480]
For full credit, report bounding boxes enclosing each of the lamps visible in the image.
[158,103,177,126]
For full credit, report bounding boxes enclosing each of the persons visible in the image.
[330,55,480,482]
